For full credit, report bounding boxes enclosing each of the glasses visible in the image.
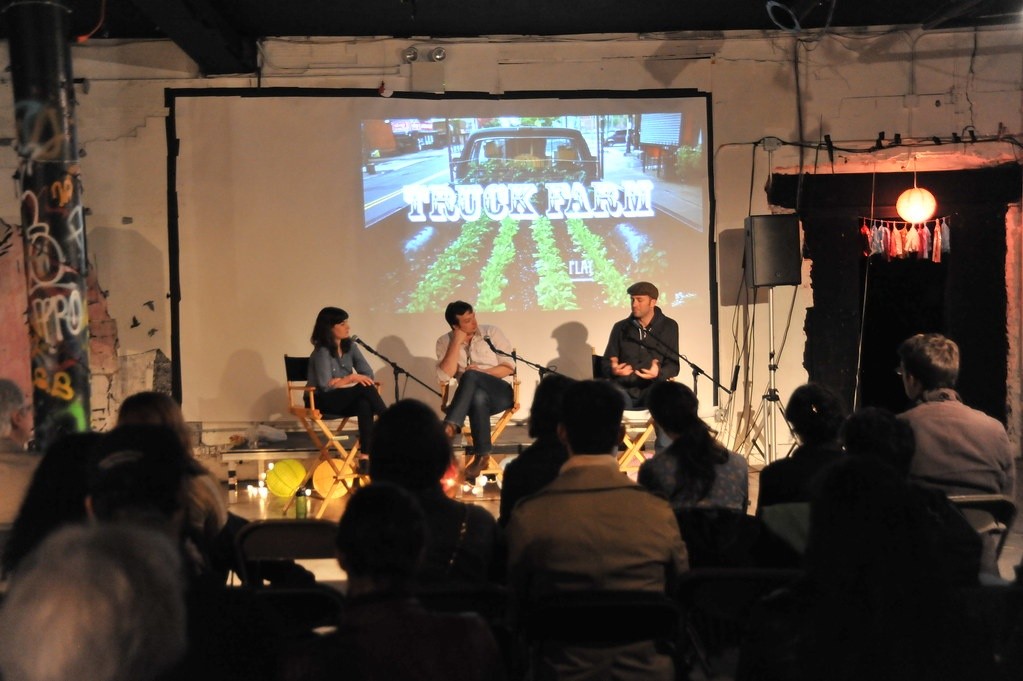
[896,367,905,374]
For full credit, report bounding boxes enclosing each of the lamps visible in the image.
[896,156,936,223]
[403,46,447,93]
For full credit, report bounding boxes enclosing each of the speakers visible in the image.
[743,214,802,287]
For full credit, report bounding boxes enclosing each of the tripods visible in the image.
[744,285,799,466]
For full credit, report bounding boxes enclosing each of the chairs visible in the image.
[280,353,384,519]
[517,589,691,681]
[441,347,521,497]
[591,346,676,473]
[664,492,1018,681]
[208,514,365,681]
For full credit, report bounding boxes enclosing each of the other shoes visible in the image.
[442,424,454,440]
[465,455,489,479]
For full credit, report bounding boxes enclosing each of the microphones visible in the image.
[351,335,373,353]
[629,316,643,331]
[483,336,497,353]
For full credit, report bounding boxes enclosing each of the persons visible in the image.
[303,307,389,474]
[602,282,680,411]
[499,381,689,681]
[0,334,1023,681]
[437,302,515,477]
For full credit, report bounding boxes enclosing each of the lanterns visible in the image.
[266,459,308,498]
[896,187,937,225]
[312,459,353,499]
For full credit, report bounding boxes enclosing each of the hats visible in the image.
[628,283,659,299]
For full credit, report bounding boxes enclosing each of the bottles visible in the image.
[296,488,307,519]
